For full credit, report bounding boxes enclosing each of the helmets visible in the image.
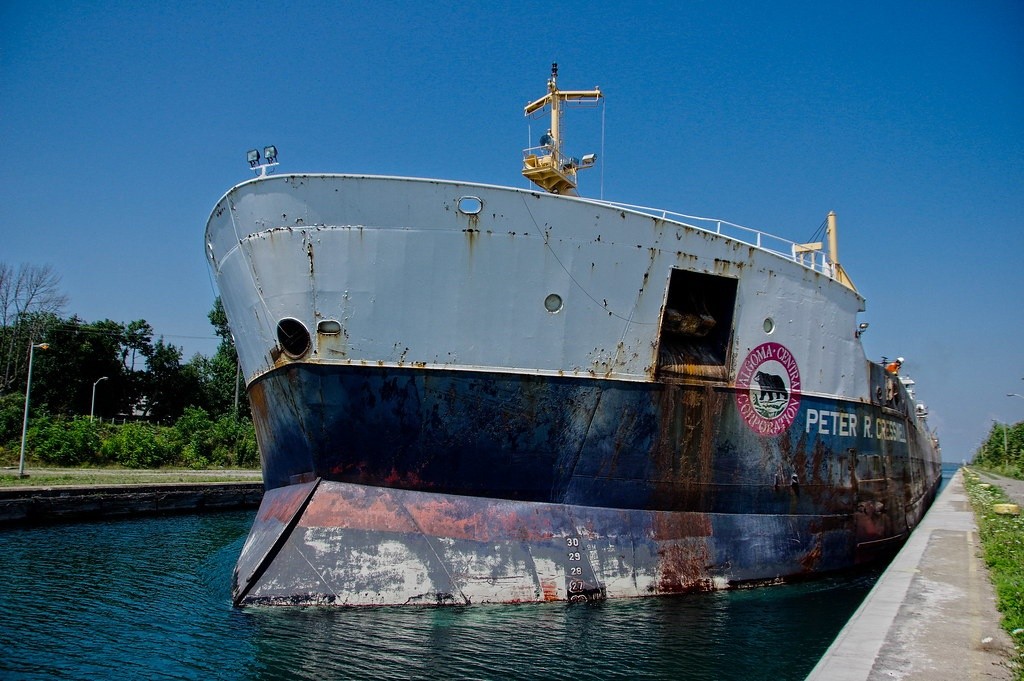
[897,357,905,364]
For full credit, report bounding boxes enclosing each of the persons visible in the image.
[886,357,904,376]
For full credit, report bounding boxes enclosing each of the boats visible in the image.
[204,59,943,610]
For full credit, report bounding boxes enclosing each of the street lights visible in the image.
[90,377,109,424]
[19,339,51,478]
[991,417,1009,472]
[1006,393,1024,399]
